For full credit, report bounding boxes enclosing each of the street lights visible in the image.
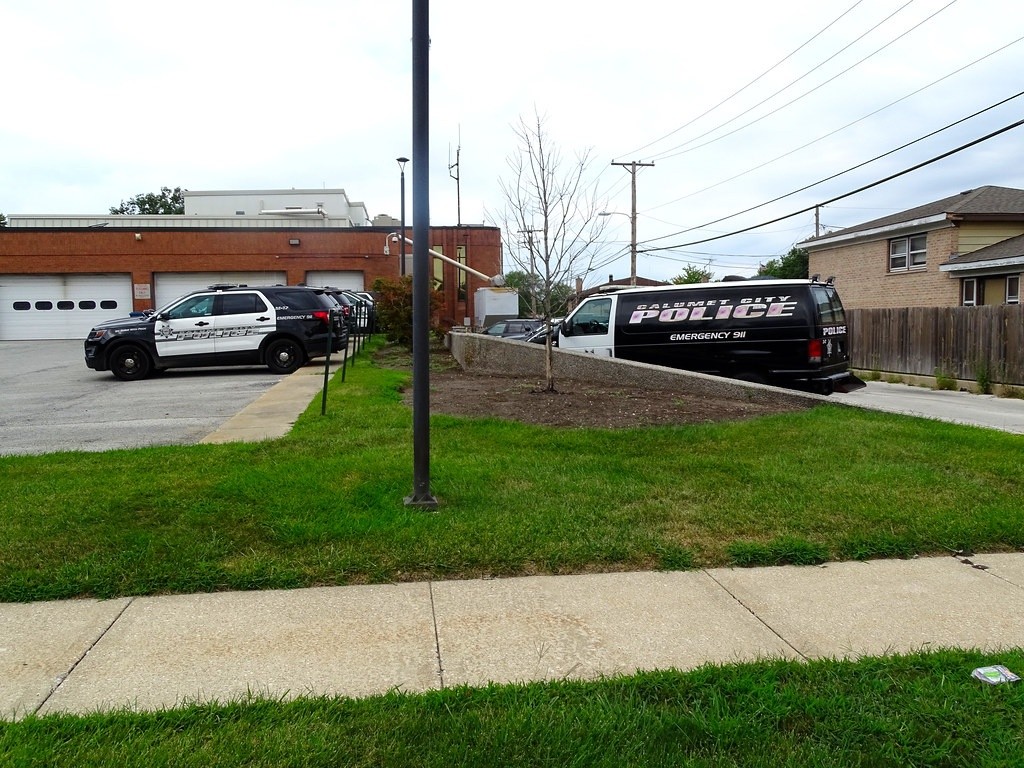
[396,158,409,277]
[599,212,636,286]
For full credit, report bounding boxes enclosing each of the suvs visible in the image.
[479,314,608,348]
[83,283,392,381]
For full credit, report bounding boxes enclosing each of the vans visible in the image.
[557,274,866,397]
